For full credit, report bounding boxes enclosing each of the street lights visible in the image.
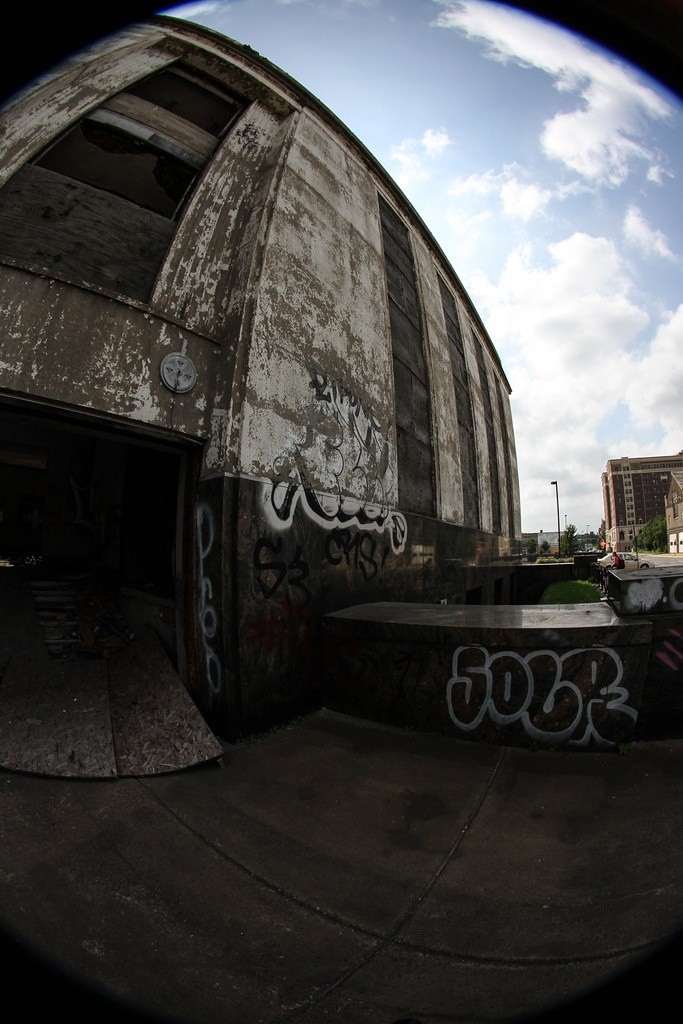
[550,481,561,557]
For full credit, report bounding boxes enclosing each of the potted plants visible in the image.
[527,538,537,562]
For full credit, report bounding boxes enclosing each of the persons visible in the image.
[611,552,621,570]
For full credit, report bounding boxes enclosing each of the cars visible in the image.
[597,552,654,570]
